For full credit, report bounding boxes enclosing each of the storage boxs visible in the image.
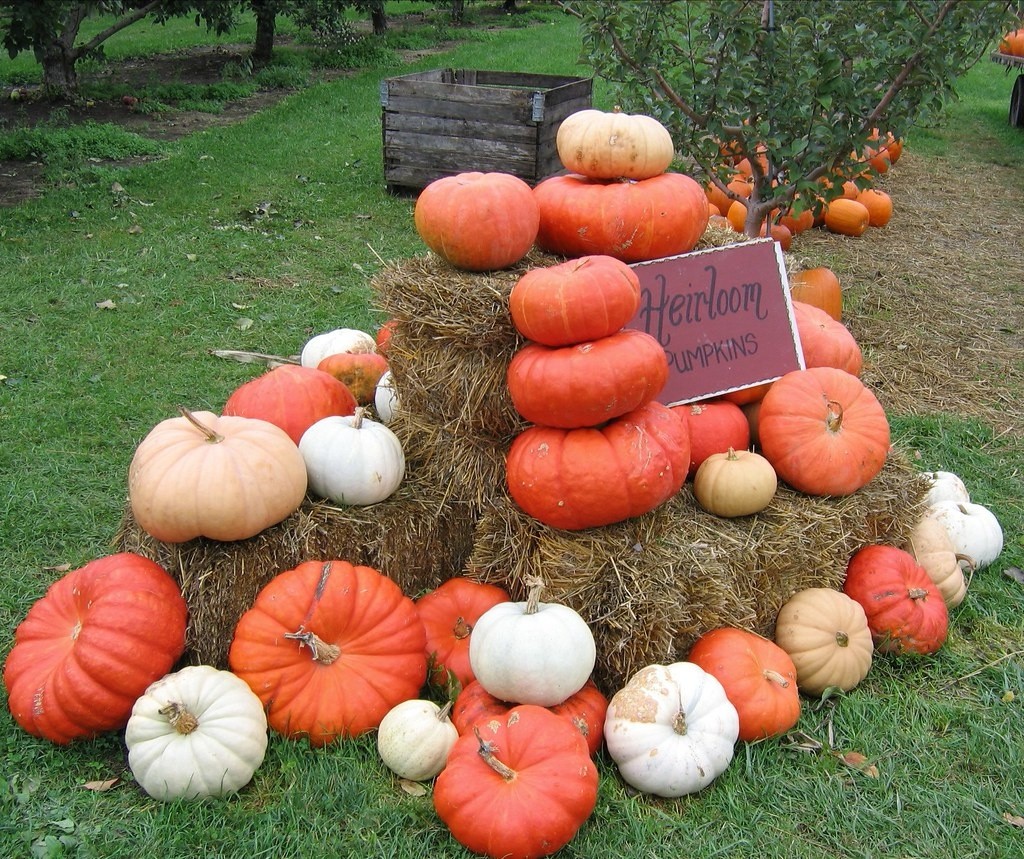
[379,67,593,199]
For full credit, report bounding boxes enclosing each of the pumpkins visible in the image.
[999,28,1024,57]
[4,116,1003,859]
